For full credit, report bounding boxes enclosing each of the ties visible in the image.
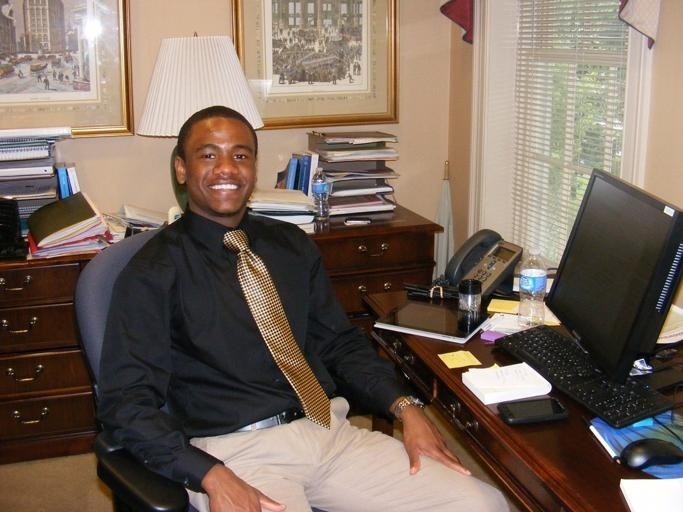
[223,229,331,429]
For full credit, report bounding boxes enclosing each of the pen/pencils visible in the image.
[583,416,622,464]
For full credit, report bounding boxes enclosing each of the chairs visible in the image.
[75,227,195,511]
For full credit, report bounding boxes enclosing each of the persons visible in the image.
[94,106,512,511]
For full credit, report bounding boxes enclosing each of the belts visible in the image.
[237,405,306,431]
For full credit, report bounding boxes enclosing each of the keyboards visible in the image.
[495,325,675,430]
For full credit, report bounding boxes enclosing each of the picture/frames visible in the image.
[0,0,135,142]
[232,0,402,131]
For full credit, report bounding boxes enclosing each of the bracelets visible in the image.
[393,394,425,424]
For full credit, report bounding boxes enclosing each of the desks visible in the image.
[360,259,681,511]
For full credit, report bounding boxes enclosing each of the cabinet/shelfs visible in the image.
[0,201,444,465]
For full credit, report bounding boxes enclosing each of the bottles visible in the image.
[456,312,482,337]
[311,166,331,220]
[516,248,549,328]
[459,279,482,312]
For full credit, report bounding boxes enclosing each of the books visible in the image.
[246,150,320,234]
[53,163,68,199]
[275,148,319,197]
[65,162,80,195]
[1,126,109,260]
[619,478,683,512]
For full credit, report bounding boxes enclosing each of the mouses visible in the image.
[621,438,683,469]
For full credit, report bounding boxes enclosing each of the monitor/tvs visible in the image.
[542,167,683,391]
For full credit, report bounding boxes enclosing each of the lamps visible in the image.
[137,31,264,141]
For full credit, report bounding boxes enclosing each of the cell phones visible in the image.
[343,218,372,225]
[497,398,569,424]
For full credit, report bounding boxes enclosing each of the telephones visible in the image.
[445,229,523,294]
